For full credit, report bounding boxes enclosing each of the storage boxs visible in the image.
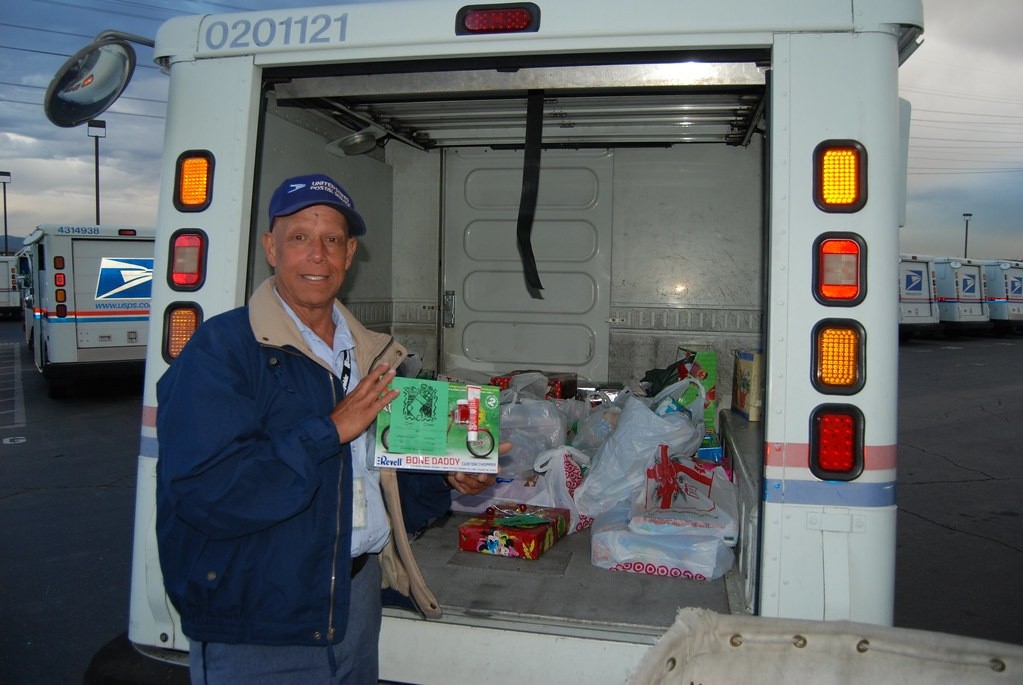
[732,348,762,421]
[697,445,724,465]
[458,503,570,559]
[491,370,587,400]
[365,376,500,476]
[674,344,717,446]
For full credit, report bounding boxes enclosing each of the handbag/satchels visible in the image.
[626,443,740,546]
[493,446,594,535]
[567,378,701,537]
[572,381,649,454]
[591,523,736,582]
[499,372,567,481]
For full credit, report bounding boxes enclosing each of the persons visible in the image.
[154,174,511,685]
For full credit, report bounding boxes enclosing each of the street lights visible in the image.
[0,171,11,256]
[962,213,973,255]
[84,120,107,225]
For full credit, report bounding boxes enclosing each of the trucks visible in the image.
[896,252,1022,345]
[0,255,22,309]
[16,223,156,379]
[41,1,925,685]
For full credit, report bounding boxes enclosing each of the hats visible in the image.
[268,174,366,239]
